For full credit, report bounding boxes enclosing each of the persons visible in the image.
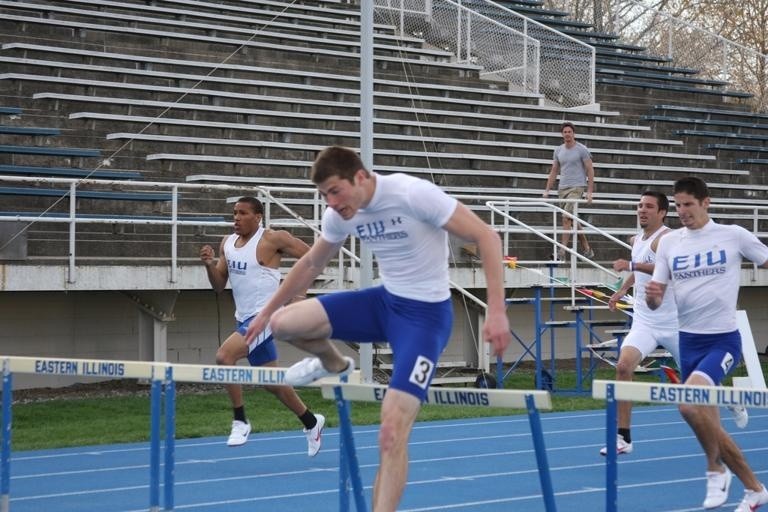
[644,177,768,511]
[244,145,510,512]
[598,192,748,455]
[543,120,595,261]
[200,195,325,457]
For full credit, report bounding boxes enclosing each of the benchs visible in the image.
[2,0,767,266]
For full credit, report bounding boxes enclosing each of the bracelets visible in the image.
[629,260,635,272]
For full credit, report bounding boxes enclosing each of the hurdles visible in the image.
[319,380,560,512]
[0,356,171,511]
[591,379,768,512]
[160,361,361,512]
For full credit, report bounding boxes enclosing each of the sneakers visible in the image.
[551,252,566,261]
[734,484,768,512]
[601,435,633,456]
[729,404,749,430]
[227,417,252,448]
[303,413,326,457]
[702,466,730,509]
[578,247,595,262]
